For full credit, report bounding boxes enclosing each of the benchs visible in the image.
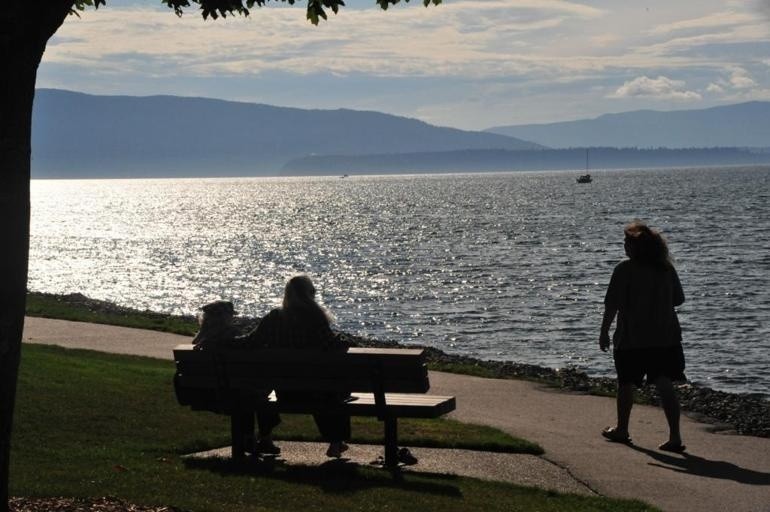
[172,342,457,469]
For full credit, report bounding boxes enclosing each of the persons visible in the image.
[191,300,280,455]
[599,223,687,452]
[192,274,358,458]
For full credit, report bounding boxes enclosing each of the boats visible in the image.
[576,148,592,183]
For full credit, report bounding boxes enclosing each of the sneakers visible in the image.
[259,442,281,454]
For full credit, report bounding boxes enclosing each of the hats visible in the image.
[202,300,239,315]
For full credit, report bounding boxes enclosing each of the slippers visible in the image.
[658,439,686,451]
[600,425,632,442]
